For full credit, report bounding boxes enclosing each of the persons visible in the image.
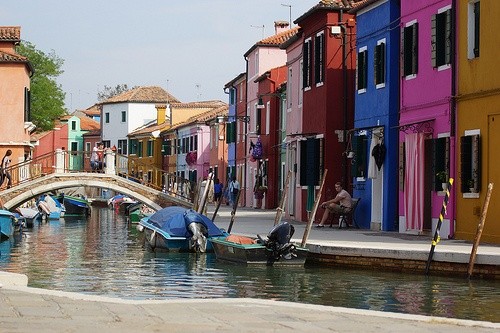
[315,182,351,228]
[90,142,106,173]
[0,150,12,189]
[170,175,262,209]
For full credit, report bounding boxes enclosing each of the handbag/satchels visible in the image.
[233,187,239,196]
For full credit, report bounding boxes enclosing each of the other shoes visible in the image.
[314,225,324,228]
[7,186,11,189]
[97,170,100,173]
[92,171,95,173]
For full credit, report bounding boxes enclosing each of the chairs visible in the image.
[329,197,361,229]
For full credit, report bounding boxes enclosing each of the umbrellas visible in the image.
[372,137,386,170]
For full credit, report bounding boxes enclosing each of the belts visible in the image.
[345,207,351,209]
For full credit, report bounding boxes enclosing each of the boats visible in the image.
[0,209,27,239]
[137,206,231,249]
[40,193,92,216]
[208,221,310,267]
